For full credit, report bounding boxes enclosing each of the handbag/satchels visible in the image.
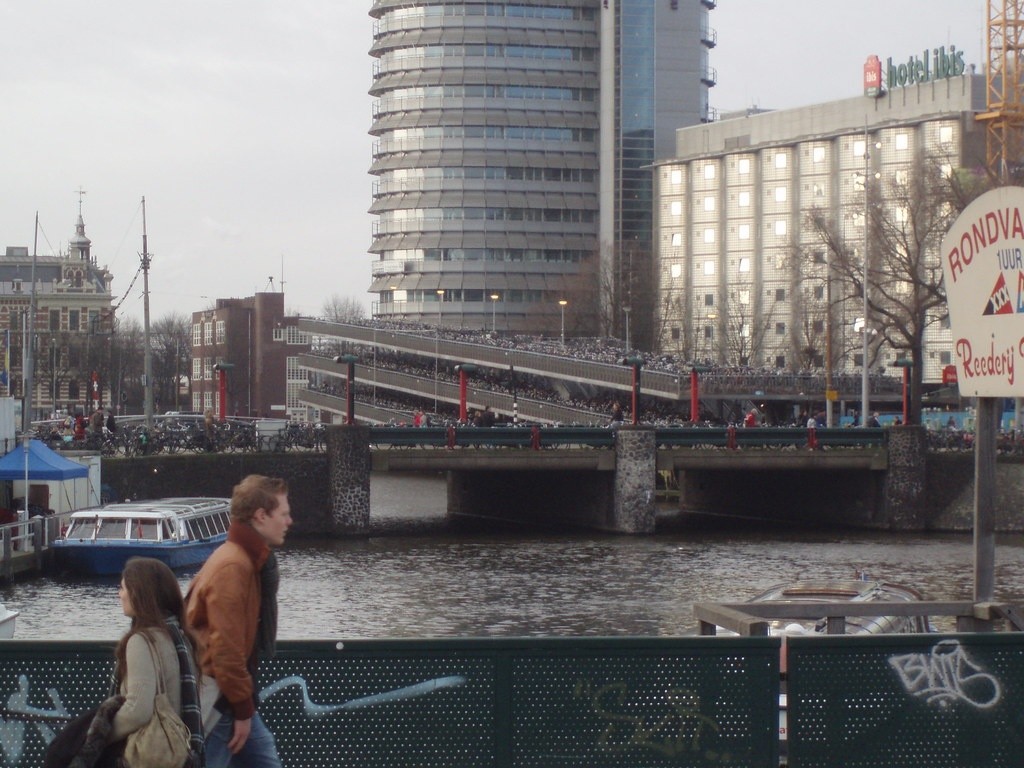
[199,674,227,741]
[113,629,192,768]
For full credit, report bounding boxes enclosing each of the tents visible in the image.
[0,439,89,538]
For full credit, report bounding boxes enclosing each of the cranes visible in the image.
[975,0,1024,190]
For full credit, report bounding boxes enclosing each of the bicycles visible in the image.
[269,420,614,453]
[33,423,264,456]
[305,346,435,381]
[733,367,905,396]
[570,344,733,392]
[313,317,441,342]
[438,366,552,401]
[925,427,1024,454]
[443,327,568,360]
[553,391,708,429]
[642,418,888,452]
[412,397,470,427]
[309,384,411,415]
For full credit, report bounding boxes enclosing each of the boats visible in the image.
[626,577,934,751]
[53,496,231,574]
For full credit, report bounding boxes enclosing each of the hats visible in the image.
[751,408,758,416]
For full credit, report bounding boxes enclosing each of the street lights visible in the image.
[490,293,499,333]
[558,299,568,344]
[437,289,445,329]
[623,306,633,351]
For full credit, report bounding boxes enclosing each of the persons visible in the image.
[849,415,859,427]
[947,416,955,429]
[182,475,292,768]
[74,413,86,440]
[203,406,215,435]
[234,409,239,420]
[466,405,495,427]
[747,408,759,427]
[608,402,624,427]
[62,412,74,436]
[797,409,827,428]
[869,412,883,448]
[108,558,206,768]
[89,406,104,433]
[894,416,902,425]
[413,409,431,428]
[106,410,115,432]
